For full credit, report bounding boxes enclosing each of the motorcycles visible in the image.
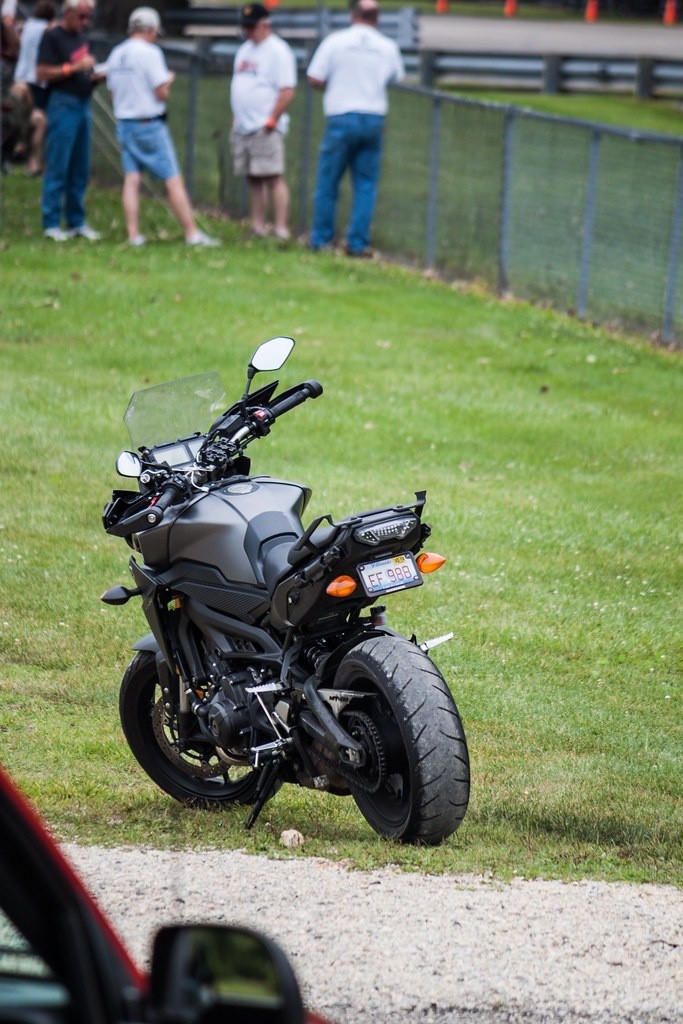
[95,336,472,845]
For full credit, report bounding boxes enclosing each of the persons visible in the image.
[0,0,57,176]
[229,3,297,242]
[36,1,105,243]
[106,7,224,247]
[296,0,406,265]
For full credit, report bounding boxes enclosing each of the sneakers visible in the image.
[71,224,100,243]
[347,246,380,261]
[185,232,222,248]
[274,228,291,239]
[41,223,69,239]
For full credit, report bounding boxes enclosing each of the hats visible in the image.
[236,1,269,27]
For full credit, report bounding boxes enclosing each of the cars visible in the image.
[3,765,327,1023]
